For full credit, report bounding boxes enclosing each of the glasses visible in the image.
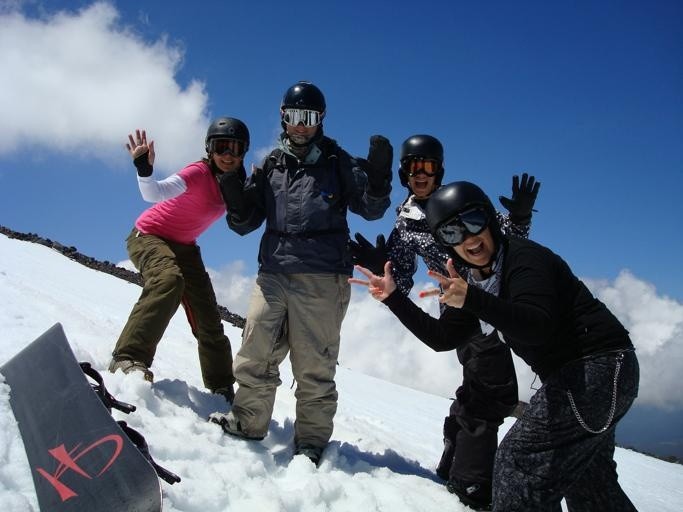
[280,107,326,128]
[206,138,247,158]
[400,158,441,178]
[436,207,488,247]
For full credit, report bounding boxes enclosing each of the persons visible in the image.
[206,79,394,468]
[416,181,645,512]
[110,114,250,407]
[341,132,544,512]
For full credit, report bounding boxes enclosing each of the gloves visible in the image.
[357,136,395,194]
[343,233,387,273]
[498,173,539,225]
[220,173,245,221]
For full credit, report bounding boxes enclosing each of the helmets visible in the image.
[281,82,325,113]
[400,134,444,164]
[207,117,250,153]
[427,182,495,247]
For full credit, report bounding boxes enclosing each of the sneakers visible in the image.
[212,385,234,404]
[295,446,321,467]
[111,356,153,382]
[208,410,263,440]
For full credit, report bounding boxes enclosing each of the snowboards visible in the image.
[0,323,180,508]
